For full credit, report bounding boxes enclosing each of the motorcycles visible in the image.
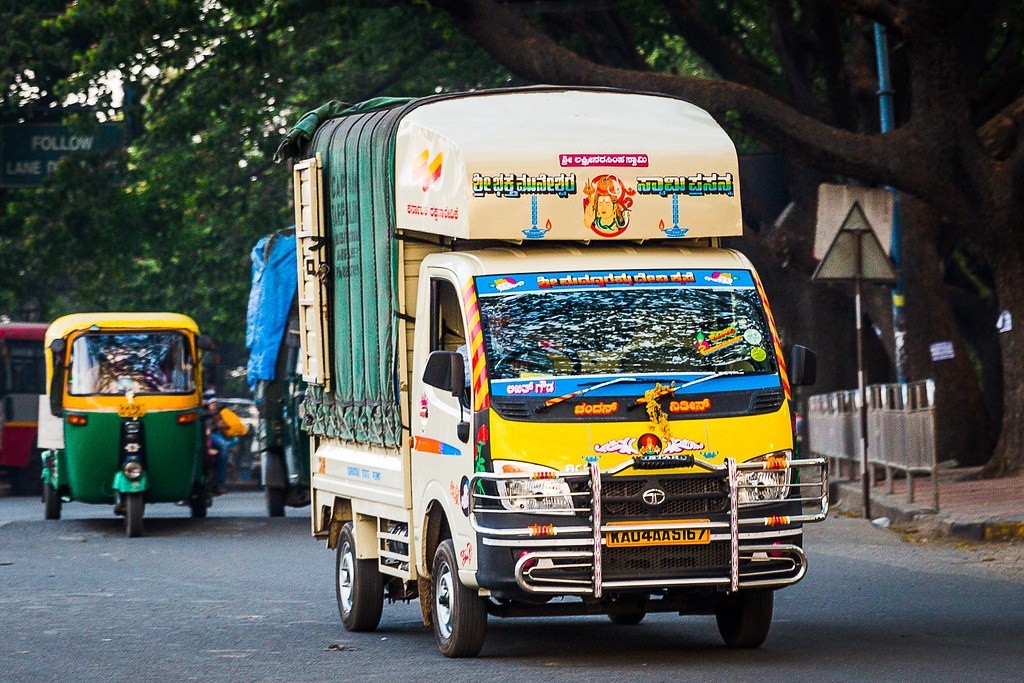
[0,315,55,496]
[220,399,264,491]
[37,311,208,537]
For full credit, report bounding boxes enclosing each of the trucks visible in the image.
[246,225,315,520]
[275,86,828,657]
